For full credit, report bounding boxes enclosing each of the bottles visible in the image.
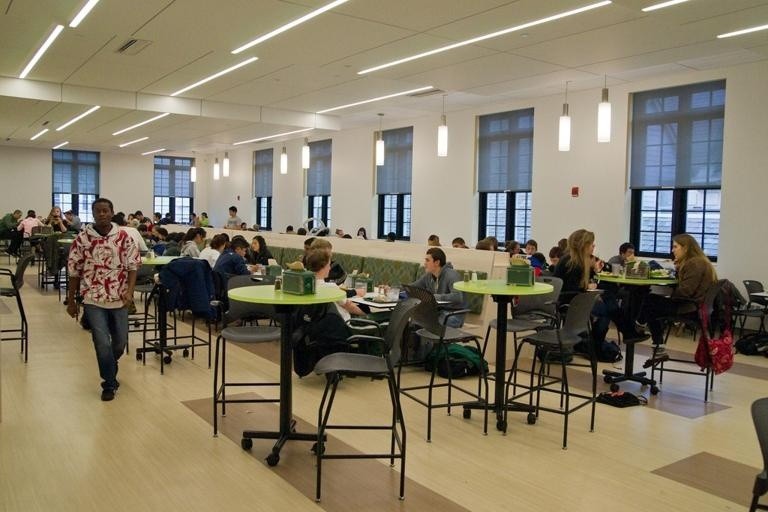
[150,249,154,259]
[471,270,477,282]
[275,276,281,290]
[620,266,623,275]
[612,263,621,275]
[464,270,470,282]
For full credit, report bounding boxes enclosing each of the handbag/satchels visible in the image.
[596,390,648,408]
[426,345,487,379]
[695,329,735,375]
[293,340,325,379]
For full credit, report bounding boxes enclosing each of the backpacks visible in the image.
[310,313,353,356]
[537,344,573,366]
[735,331,768,358]
[598,340,623,363]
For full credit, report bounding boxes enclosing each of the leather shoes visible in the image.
[622,334,650,343]
[643,354,669,369]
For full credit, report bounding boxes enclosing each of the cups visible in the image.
[256,264,262,271]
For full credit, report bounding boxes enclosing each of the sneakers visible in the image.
[100,378,120,401]
[29,258,36,266]
[5,247,19,257]
[675,321,685,337]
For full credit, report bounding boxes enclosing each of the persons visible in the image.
[67,198,141,401]
[644,234,719,368]
[608,243,635,266]
[408,247,464,310]
[111,205,274,290]
[428,235,569,273]
[285,225,396,242]
[302,237,372,328]
[0,206,81,265]
[551,229,651,350]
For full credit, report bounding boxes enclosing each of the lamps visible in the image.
[436,93,447,157]
[280,144,288,174]
[558,80,572,151]
[597,73,612,142]
[212,156,220,181]
[223,151,230,180]
[301,133,311,169]
[190,157,197,186]
[375,113,385,168]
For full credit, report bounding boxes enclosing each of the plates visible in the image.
[652,275,671,278]
[596,272,615,277]
